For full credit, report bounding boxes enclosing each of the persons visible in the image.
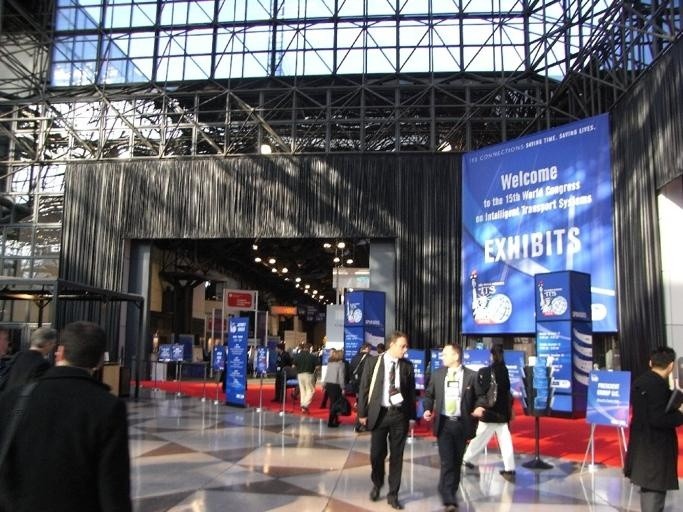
[423,343,487,512]
[271,344,385,433]
[358,330,417,509]
[0,323,135,512]
[462,344,516,482]
[624,347,683,511]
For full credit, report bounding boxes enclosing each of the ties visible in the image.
[390,362,395,396]
[448,372,456,413]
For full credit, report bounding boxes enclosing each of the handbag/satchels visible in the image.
[333,399,352,415]
[486,367,498,407]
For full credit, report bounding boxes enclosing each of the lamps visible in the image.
[250,241,353,305]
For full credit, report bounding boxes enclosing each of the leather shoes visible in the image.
[372,487,379,501]
[388,497,401,509]
